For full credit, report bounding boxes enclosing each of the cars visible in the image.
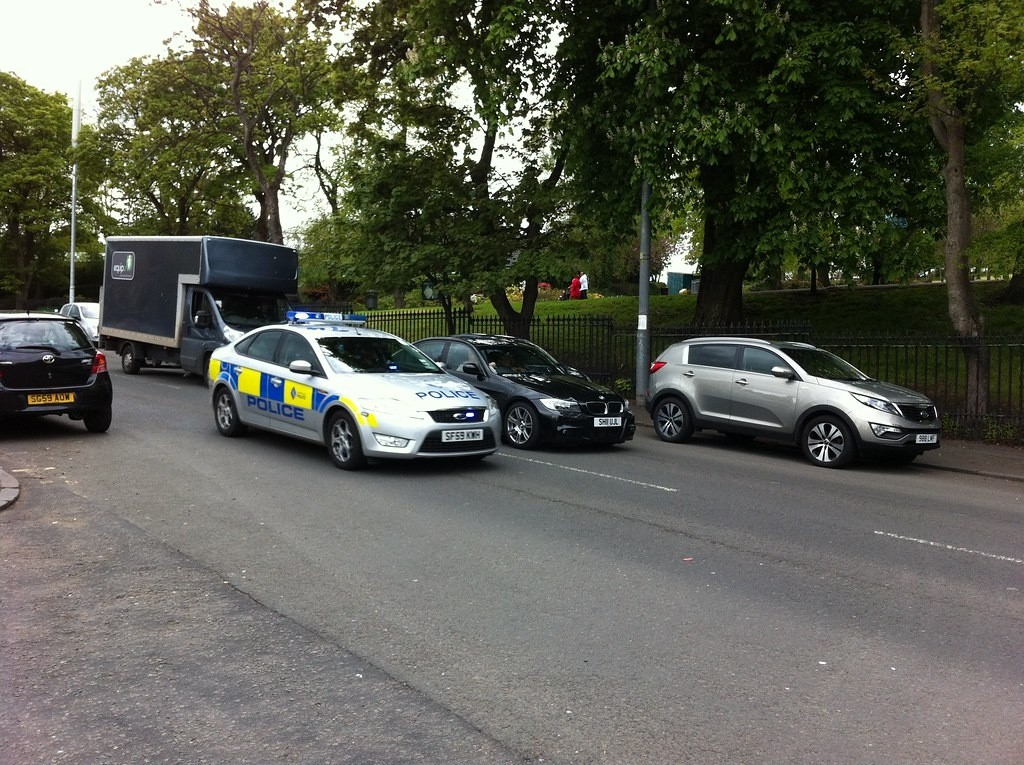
[54,302,101,348]
[385,332,638,451]
[0,308,114,433]
[205,309,502,473]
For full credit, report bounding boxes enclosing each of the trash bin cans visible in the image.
[364,292,379,310]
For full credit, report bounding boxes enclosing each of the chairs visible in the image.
[20,325,49,345]
[487,351,511,367]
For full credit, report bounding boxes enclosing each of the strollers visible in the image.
[557,287,571,301]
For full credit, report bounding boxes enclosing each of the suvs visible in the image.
[644,337,943,470]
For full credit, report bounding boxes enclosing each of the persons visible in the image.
[568,272,588,300]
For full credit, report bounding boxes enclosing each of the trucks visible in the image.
[95,235,301,388]
